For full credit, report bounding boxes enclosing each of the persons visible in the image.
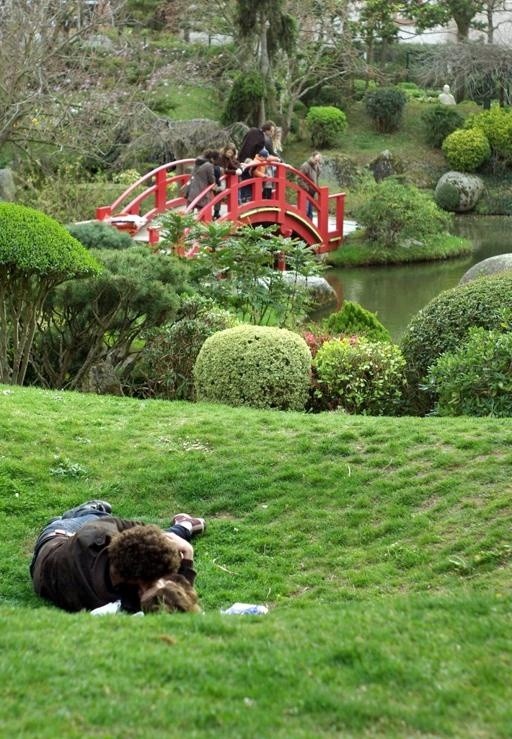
[177,119,322,222]
[439,84,456,106]
[138,512,206,618]
[31,500,196,614]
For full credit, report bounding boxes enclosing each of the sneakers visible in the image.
[80,501,112,514]
[172,512,206,536]
